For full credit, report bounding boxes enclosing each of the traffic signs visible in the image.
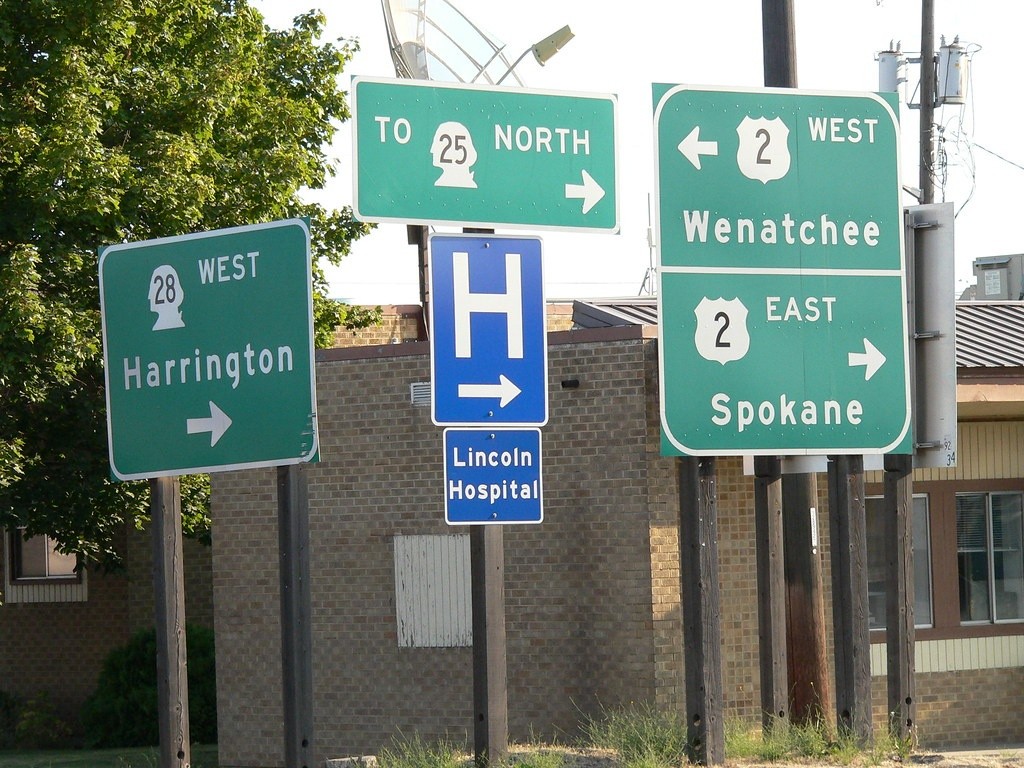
[351,75,623,233]
[99,216,323,482]
[427,226,550,426]
[656,267,915,455]
[649,82,906,273]
